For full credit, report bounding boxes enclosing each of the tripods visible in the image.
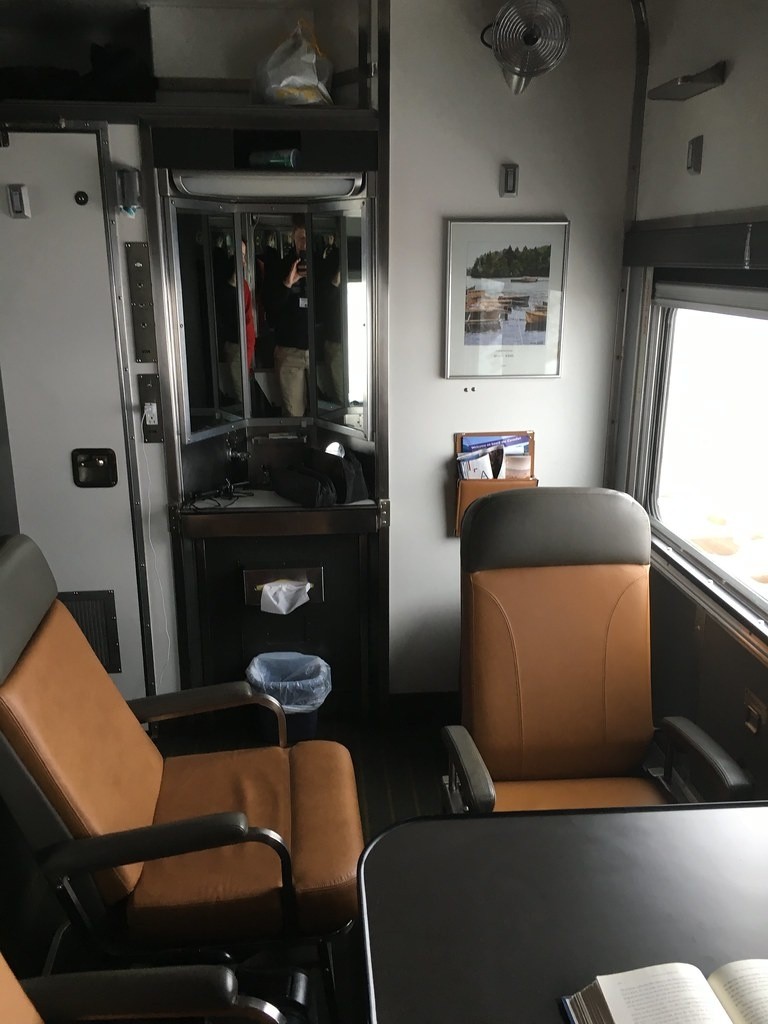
[189,480,255,503]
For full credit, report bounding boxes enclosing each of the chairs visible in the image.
[0,534,366,1023]
[441,485,757,812]
[0,950,287,1023]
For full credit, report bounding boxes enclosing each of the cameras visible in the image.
[297,261,307,273]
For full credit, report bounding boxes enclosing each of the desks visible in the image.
[178,500,378,686]
[359,799,768,1024]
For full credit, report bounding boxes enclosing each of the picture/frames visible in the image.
[445,220,571,378]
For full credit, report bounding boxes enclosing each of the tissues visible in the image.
[243,566,325,615]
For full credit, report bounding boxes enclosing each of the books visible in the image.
[562,958,768,1024]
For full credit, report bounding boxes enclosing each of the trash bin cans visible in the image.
[244,650,328,746]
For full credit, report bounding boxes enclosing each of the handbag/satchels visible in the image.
[256,17,333,106]
[272,445,368,507]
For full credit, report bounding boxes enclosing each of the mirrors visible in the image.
[165,197,373,447]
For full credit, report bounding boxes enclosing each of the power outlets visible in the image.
[144,402,159,426]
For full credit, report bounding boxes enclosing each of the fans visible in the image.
[488,0,573,94]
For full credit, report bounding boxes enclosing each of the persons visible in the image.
[201,214,345,417]
